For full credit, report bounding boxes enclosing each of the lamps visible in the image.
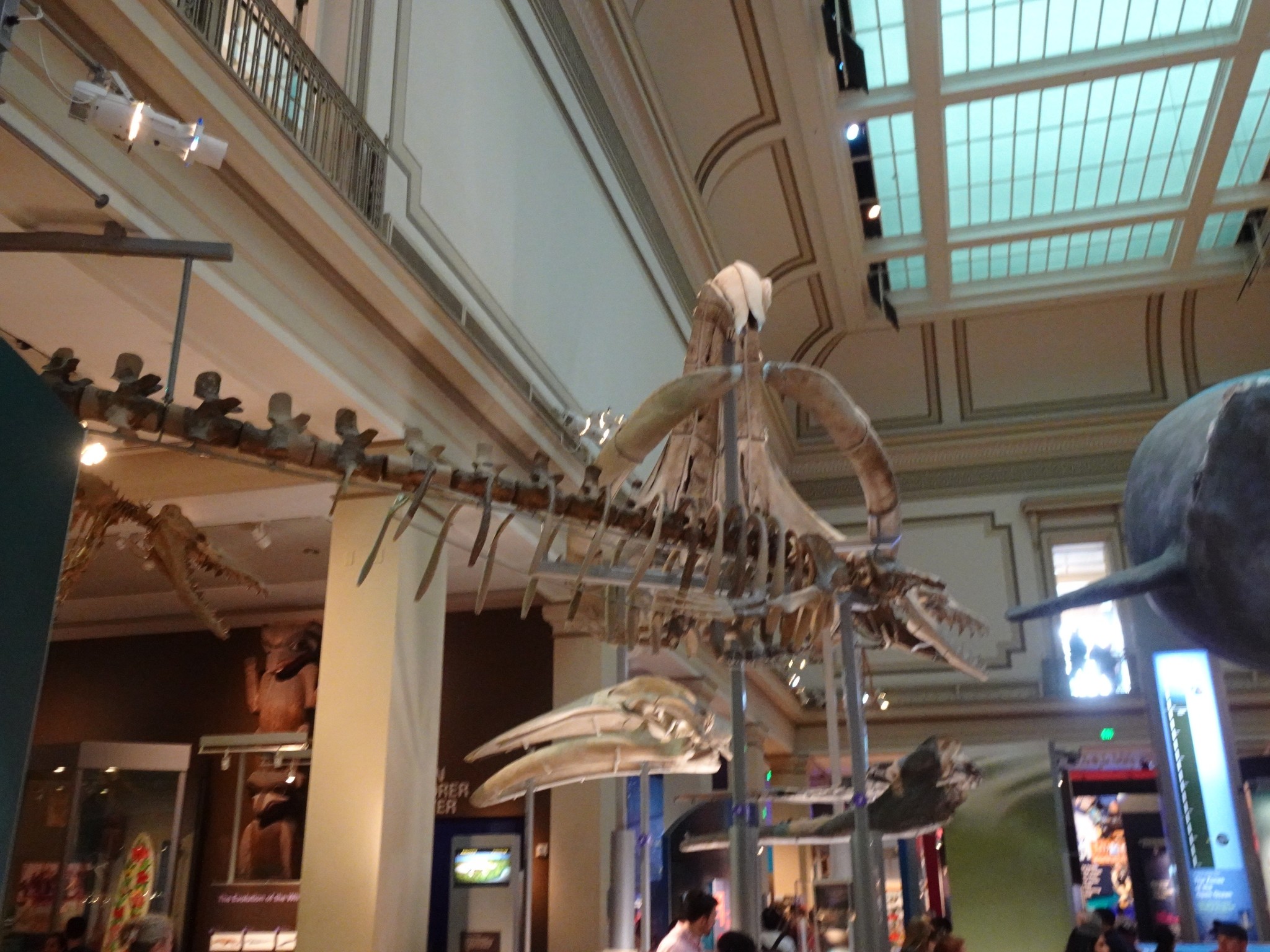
[272,754,284,767]
[784,657,811,706]
[572,407,624,446]
[221,754,231,771]
[69,79,230,172]
[874,688,890,711]
[861,200,882,220]
[285,760,301,784]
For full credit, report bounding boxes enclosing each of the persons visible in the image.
[655,891,718,952]
[717,899,965,952]
[1065,907,1175,952]
[1208,919,1248,952]
